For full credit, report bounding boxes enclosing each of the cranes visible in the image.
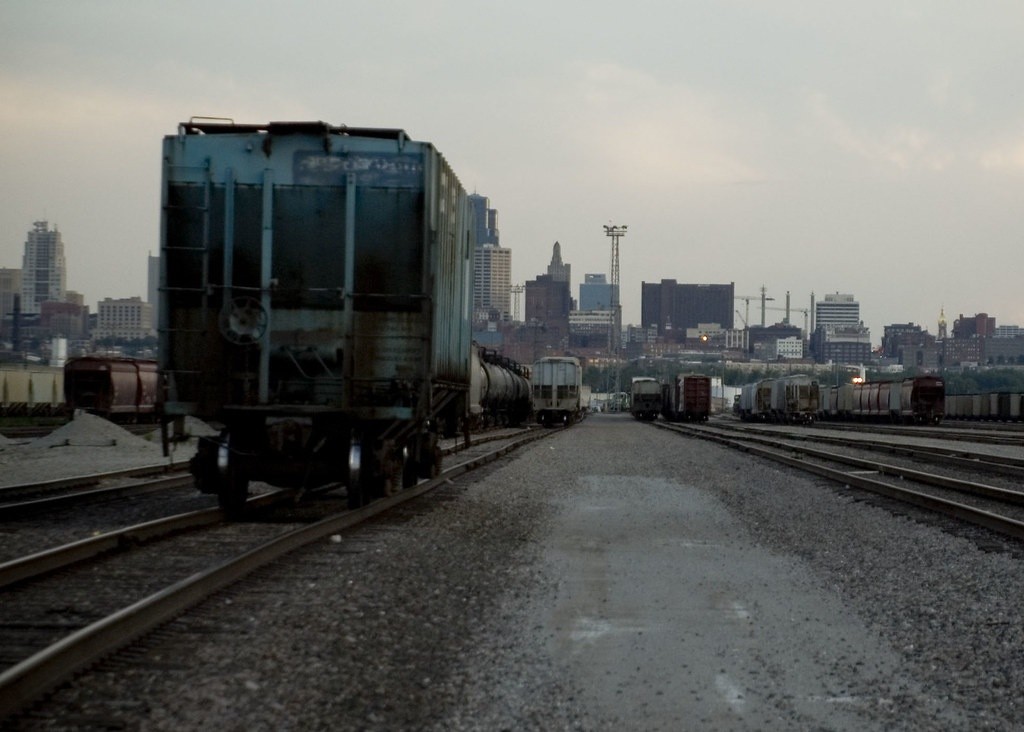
[734,284,775,327]
[733,298,749,328]
[765,290,806,324]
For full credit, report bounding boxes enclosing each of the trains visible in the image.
[666,373,710,424]
[630,376,662,423]
[532,355,592,428]
[61,352,157,425]
[733,373,821,426]
[153,118,533,510]
[816,375,947,425]
